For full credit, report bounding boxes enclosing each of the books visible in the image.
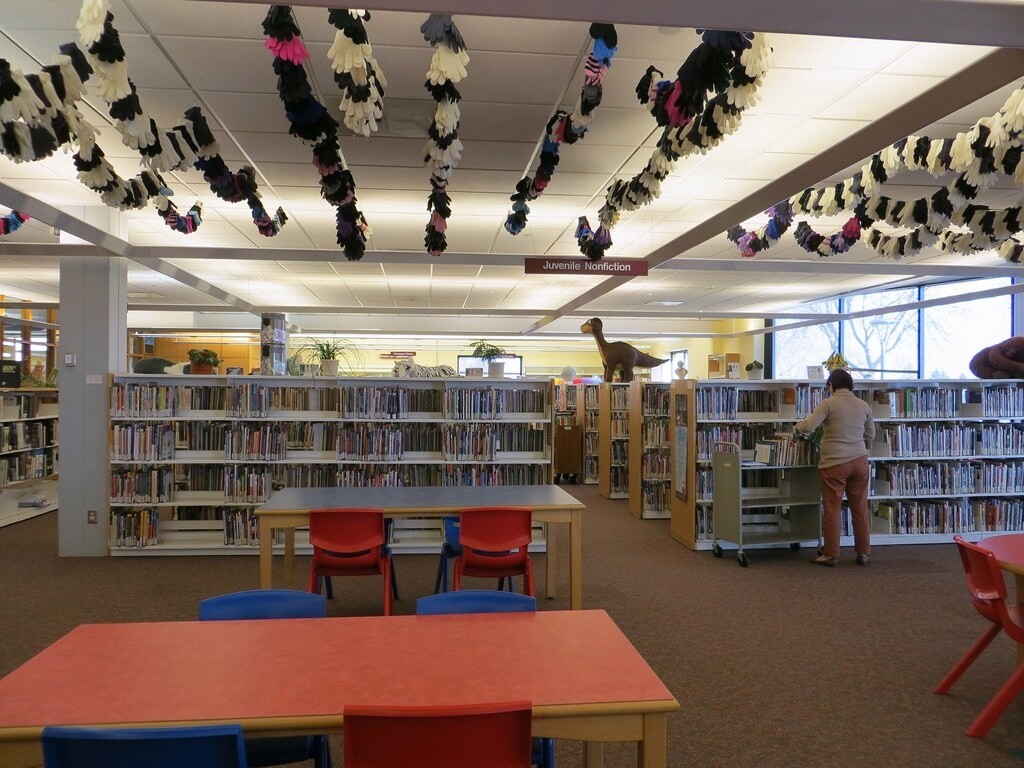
[610,386,629,493]
[873,384,1024,535]
[696,388,795,466]
[554,386,577,426]
[728,362,740,378]
[821,461,876,536]
[585,385,600,479]
[795,386,831,466]
[807,366,823,379]
[18,490,52,508]
[465,368,483,377]
[109,384,553,548]
[695,468,714,541]
[642,387,672,512]
[0,391,59,486]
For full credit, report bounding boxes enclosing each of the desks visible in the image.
[975,533,1024,603]
[254,485,586,611]
[0,607,682,768]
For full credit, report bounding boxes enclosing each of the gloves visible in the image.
[505,21,768,260]
[726,88,1024,268]
[0,0,259,235]
[416,13,471,258]
[259,4,388,261]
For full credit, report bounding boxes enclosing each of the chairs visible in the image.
[343,699,533,768]
[416,589,556,768]
[434,517,515,594]
[41,723,247,768]
[931,533,1024,739]
[307,508,392,616]
[452,507,535,597]
[318,518,400,602]
[198,589,332,768]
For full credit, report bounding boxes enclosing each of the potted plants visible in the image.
[745,360,764,380]
[468,338,508,380]
[187,348,220,375]
[290,335,369,386]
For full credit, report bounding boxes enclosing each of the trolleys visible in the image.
[712,442,827,568]
[555,411,582,483]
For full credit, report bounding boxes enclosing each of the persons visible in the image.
[796,368,878,567]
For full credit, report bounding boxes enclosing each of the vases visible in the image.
[829,364,846,374]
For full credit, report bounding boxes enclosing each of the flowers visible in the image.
[821,350,851,373]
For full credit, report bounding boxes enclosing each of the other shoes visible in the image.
[809,557,837,567]
[856,555,867,565]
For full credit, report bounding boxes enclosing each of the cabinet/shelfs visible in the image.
[558,382,576,411]
[576,383,600,486]
[711,440,824,569]
[629,380,670,520]
[109,372,556,557]
[670,377,1024,552]
[0,388,59,528]
[597,381,630,501]
